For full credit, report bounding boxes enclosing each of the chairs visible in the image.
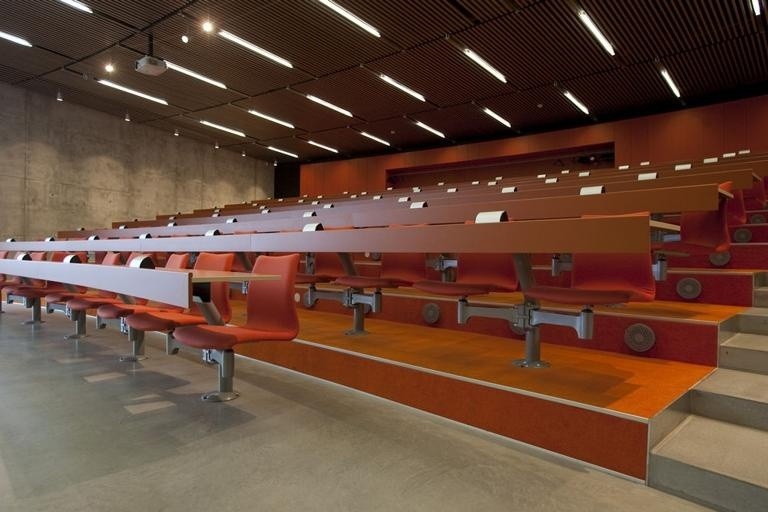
[1,151,768,404]
